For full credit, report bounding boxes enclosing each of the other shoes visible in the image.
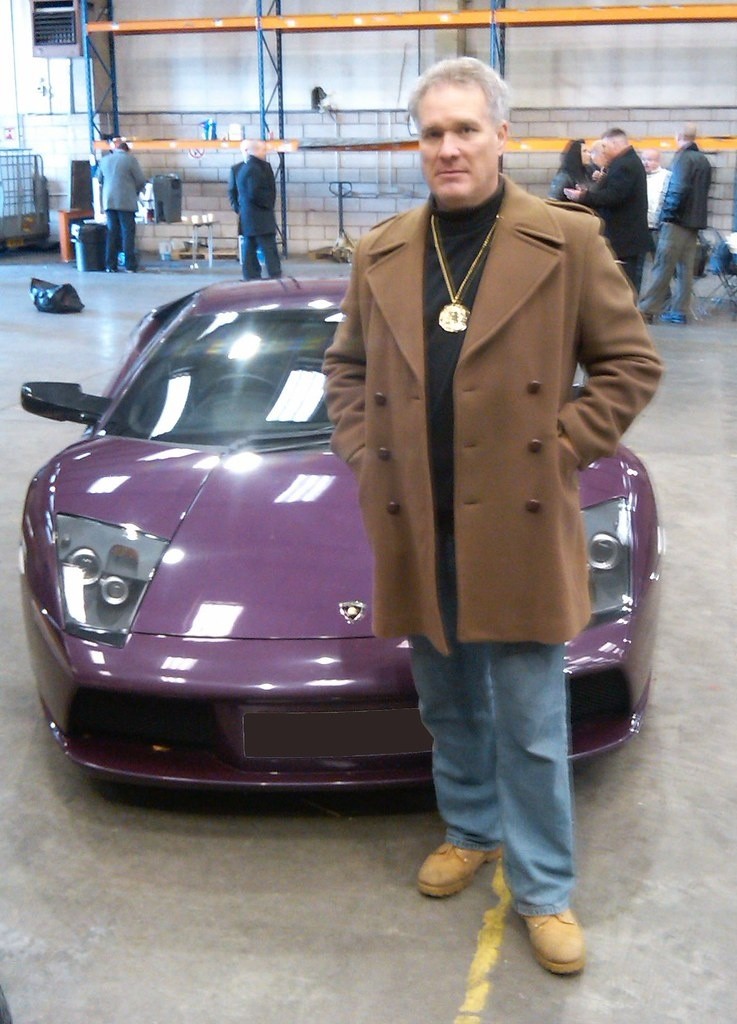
[521,906,587,975]
[106,265,135,273]
[643,311,687,324]
[417,842,502,896]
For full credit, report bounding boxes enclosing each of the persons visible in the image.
[552,128,656,312]
[322,54,670,976]
[633,149,672,258]
[229,138,282,280]
[638,123,719,326]
[97,143,145,269]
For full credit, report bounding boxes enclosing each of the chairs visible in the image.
[698,225,737,320]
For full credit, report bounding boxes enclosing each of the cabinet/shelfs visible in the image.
[77,0,737,272]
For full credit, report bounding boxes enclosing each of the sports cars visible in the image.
[16,272,660,813]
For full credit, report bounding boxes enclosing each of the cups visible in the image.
[191,213,214,224]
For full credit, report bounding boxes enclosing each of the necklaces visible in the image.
[430,211,501,335]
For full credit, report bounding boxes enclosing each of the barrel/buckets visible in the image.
[69,223,107,272]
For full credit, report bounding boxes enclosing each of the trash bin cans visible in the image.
[71,223,108,272]
